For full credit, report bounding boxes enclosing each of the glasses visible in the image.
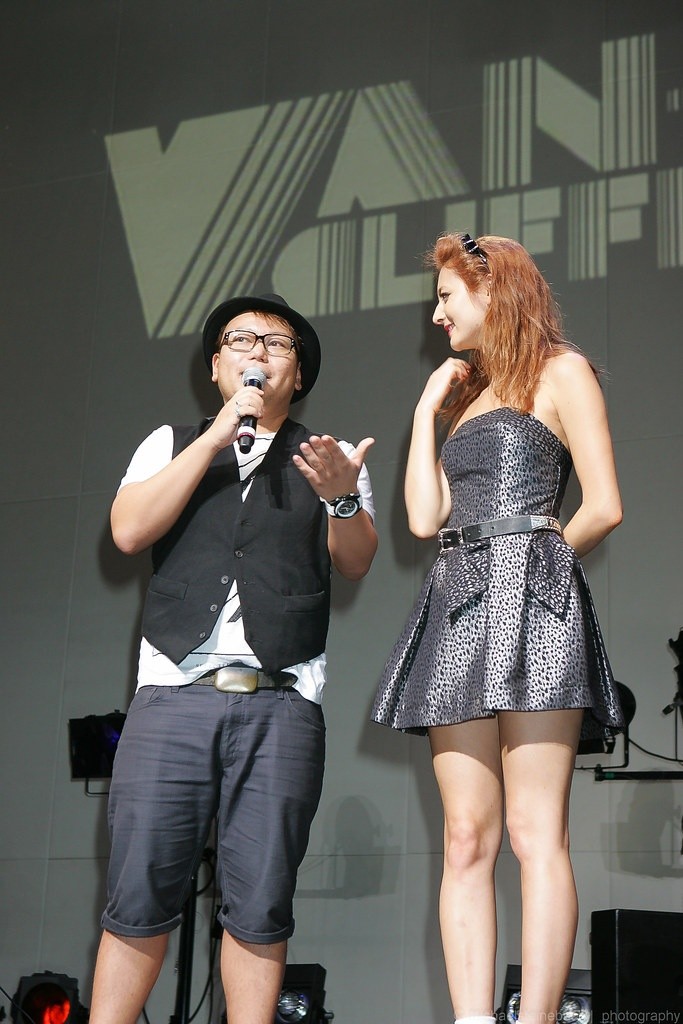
[219,329,300,356]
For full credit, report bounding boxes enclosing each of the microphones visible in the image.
[237,367,266,454]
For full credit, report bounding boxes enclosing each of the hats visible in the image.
[202,293,320,404]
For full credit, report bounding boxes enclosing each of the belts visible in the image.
[192,666,297,693]
[437,514,562,553]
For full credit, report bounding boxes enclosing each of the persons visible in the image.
[83,289,378,1023]
[374,230,624,1024]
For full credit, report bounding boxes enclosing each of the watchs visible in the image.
[326,489,364,519]
